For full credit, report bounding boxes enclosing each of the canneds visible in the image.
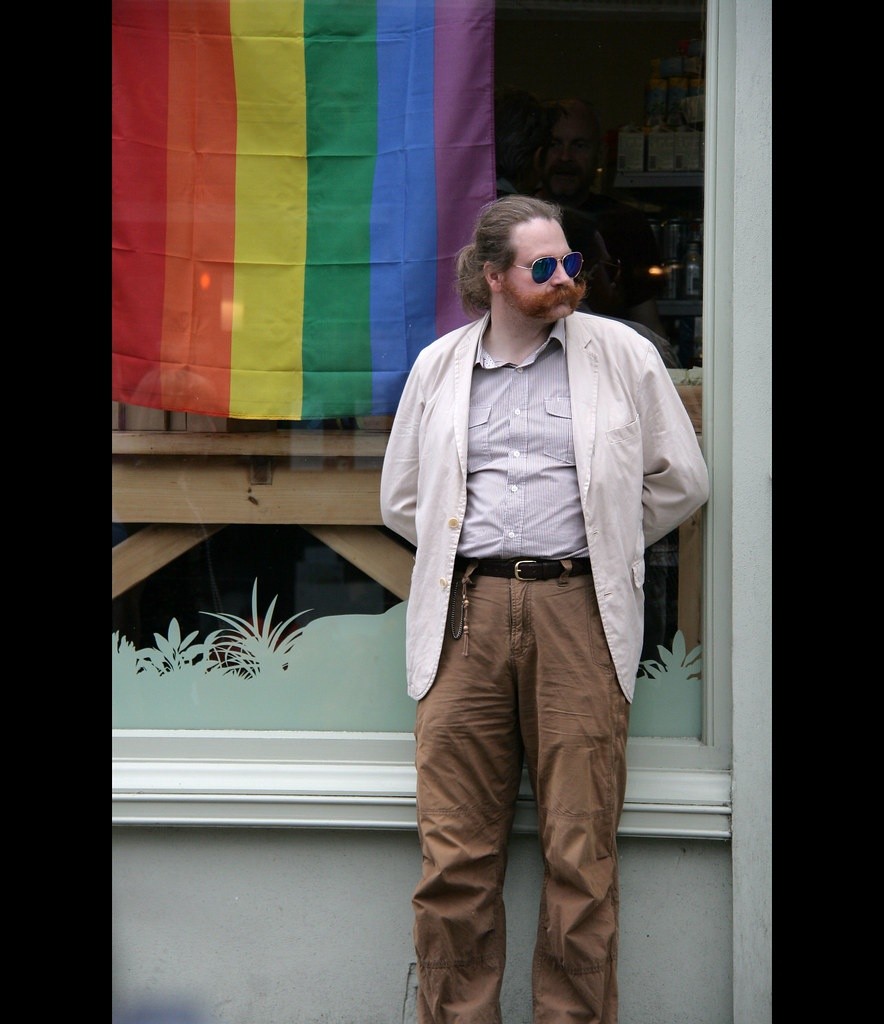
[647,217,701,301]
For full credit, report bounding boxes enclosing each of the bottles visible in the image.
[684,244,702,300]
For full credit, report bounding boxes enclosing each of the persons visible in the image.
[532,97,670,344]
[381,195,708,1024]
[494,82,612,315]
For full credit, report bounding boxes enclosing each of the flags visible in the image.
[112,0,494,420]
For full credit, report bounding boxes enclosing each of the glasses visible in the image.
[505,252,584,285]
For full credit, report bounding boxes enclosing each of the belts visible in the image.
[457,556,591,582]
